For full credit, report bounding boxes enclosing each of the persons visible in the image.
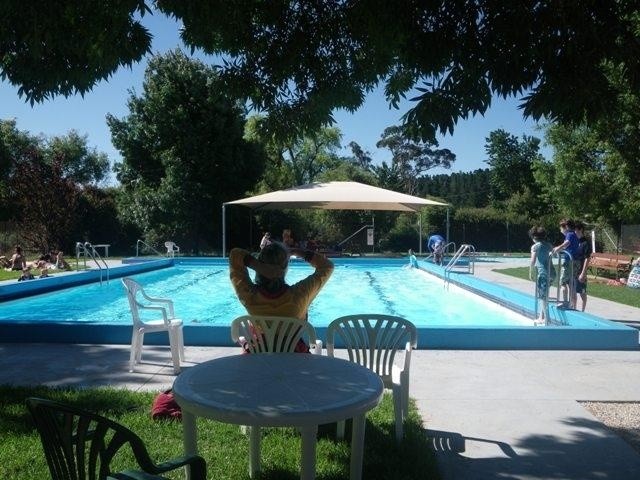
[525,224,559,327]
[281,228,318,249]
[407,248,419,269]
[572,219,593,312]
[226,242,335,355]
[259,232,273,251]
[553,218,582,312]
[0,246,69,282]
[425,232,447,265]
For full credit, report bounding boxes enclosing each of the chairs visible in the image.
[164,241,180,258]
[229,314,320,435]
[325,314,419,442]
[25,393,208,479]
[120,275,187,375]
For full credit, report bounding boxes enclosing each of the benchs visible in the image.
[583,251,634,284]
[308,246,344,259]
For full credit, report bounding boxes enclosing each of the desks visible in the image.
[172,351,387,479]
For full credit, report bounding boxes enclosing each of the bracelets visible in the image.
[303,249,314,263]
